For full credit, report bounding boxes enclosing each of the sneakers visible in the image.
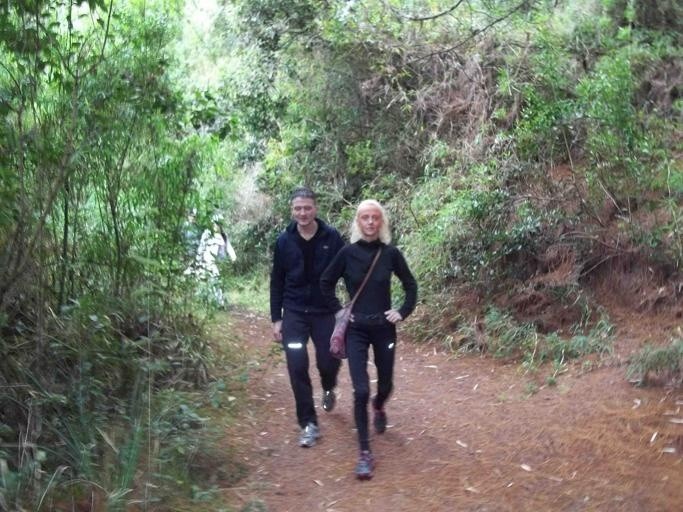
[373,407,386,433]
[300,424,318,447]
[322,390,334,410]
[357,450,373,478]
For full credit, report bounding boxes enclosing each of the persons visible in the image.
[319,200,417,480]
[197,214,237,311]
[269,188,345,448]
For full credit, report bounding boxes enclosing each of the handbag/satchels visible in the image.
[330,303,352,359]
